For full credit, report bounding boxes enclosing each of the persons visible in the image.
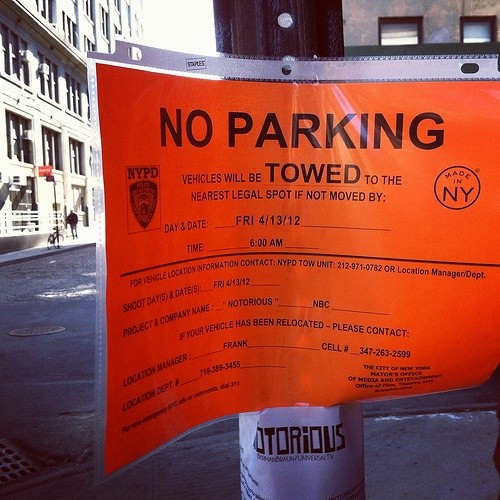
[67,209,80,240]
